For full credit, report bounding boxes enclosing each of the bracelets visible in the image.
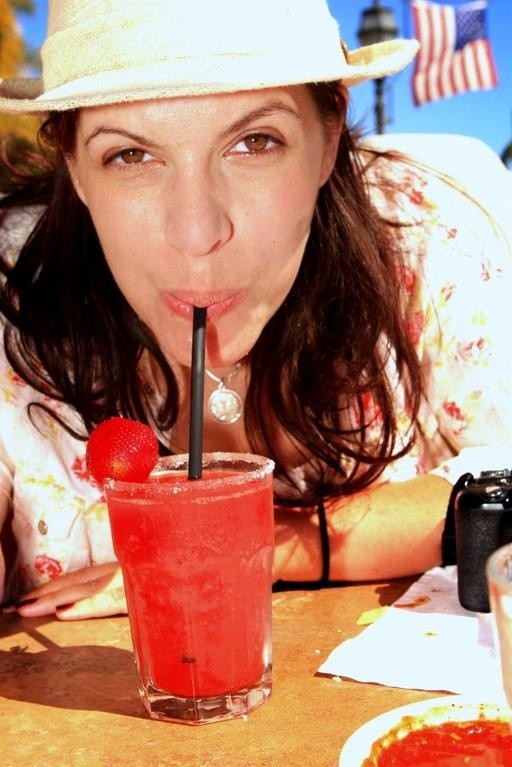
[317,495,332,584]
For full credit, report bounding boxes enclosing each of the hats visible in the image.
[0,1,420,112]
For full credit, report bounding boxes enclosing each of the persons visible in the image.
[0,0,512,623]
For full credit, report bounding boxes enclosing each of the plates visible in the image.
[339,698,512,767]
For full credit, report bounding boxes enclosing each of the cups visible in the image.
[101,451,276,726]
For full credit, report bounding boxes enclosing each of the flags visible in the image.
[411,0,499,106]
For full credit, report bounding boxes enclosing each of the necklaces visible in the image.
[205,362,243,425]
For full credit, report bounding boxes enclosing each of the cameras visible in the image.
[453,470,512,614]
